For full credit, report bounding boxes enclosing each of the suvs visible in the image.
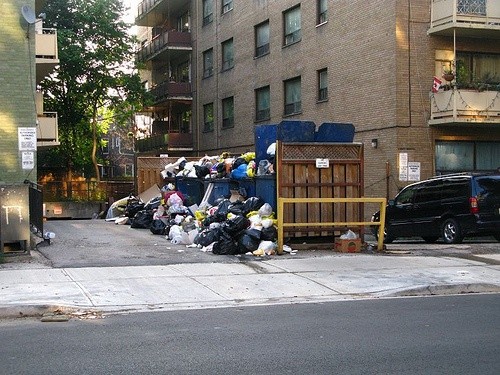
[370,169,500,244]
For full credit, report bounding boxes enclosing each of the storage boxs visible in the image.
[335,238,362,253]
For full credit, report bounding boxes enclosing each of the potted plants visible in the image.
[443,69,454,82]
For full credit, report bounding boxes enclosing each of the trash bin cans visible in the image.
[165,176,203,208]
[230,177,256,199]
[204,177,230,206]
[254,174,277,209]
